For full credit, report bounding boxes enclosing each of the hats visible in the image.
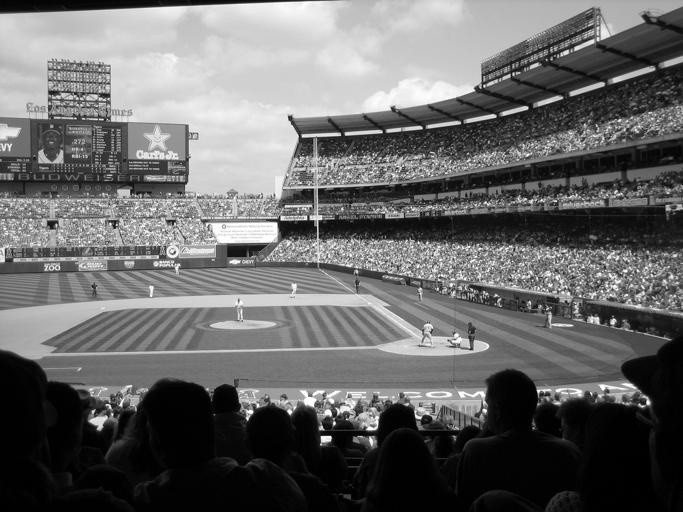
[621,334,683,406]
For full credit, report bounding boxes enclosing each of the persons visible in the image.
[148,283,155,298]
[90,281,98,297]
[37,124,64,164]
[1,189,274,264]
[174,265,180,278]
[235,297,244,322]
